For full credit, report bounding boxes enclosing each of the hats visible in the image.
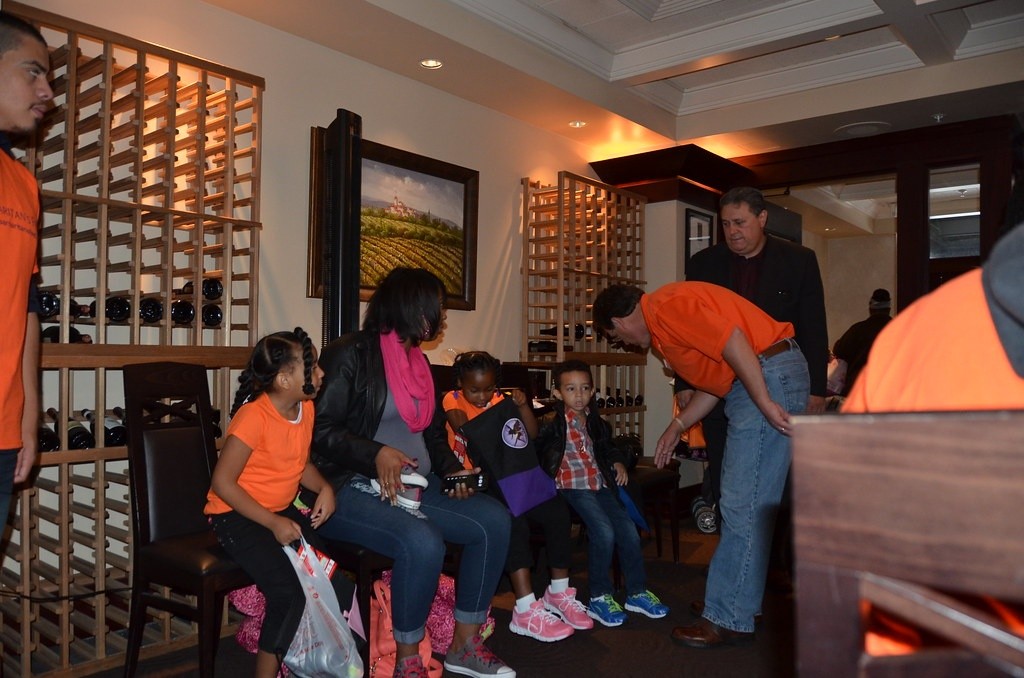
[872,289,891,302]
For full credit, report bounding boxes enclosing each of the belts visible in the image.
[762,338,799,361]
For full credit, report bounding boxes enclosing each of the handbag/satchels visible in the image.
[458,395,558,518]
[281,534,365,678]
[612,473,652,533]
[369,579,444,678]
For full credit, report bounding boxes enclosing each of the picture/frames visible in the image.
[305,127,478,311]
[684,207,713,273]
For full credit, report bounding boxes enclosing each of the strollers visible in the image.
[673,390,826,534]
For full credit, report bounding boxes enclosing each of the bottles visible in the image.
[38,422,59,452]
[37,291,59,319]
[87,296,131,322]
[172,299,194,323]
[47,407,95,450]
[82,408,128,446]
[171,278,222,300]
[528,340,573,353]
[586,326,602,341]
[42,326,91,343]
[140,298,162,323]
[539,323,584,339]
[59,298,90,319]
[201,304,222,326]
[616,388,643,406]
[111,407,127,426]
[595,387,616,408]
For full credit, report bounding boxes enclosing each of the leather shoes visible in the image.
[672,617,754,649]
[689,599,764,633]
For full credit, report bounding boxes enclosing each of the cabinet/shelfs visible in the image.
[0,0,268,678]
[521,170,646,458]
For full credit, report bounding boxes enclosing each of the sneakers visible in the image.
[542,585,594,630]
[624,590,671,619]
[586,594,628,628]
[444,636,516,678]
[392,655,430,678]
[509,597,574,643]
[401,462,428,491]
[370,478,422,510]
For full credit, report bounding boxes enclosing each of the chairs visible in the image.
[125,361,680,678]
[788,412,1024,678]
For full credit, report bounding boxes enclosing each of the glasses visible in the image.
[611,329,623,342]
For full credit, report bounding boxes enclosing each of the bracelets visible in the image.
[674,417,685,433]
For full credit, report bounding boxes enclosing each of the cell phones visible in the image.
[440,472,488,495]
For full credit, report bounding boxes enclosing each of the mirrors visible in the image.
[926,159,982,260]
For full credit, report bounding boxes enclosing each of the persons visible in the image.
[673,186,828,598]
[832,289,895,396]
[535,359,669,627]
[0,10,53,566]
[589,280,811,650]
[204,327,356,678]
[441,351,594,643]
[299,265,517,678]
[837,220,1024,655]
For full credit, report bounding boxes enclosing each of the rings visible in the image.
[381,484,385,487]
[781,427,784,433]
[385,483,389,485]
